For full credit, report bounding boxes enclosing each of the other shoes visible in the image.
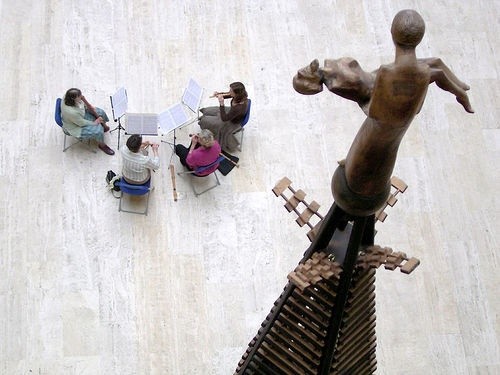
[98,143,114,155]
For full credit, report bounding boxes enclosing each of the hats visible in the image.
[198,129,214,147]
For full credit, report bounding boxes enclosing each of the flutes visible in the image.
[140,142,158,148]
[208,91,230,99]
[189,135,199,138]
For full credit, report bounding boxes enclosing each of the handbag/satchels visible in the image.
[106,171,123,199]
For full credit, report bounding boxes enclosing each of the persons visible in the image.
[61,88,115,155]
[293,56,475,114]
[331,10,431,218]
[175,129,221,177]
[198,82,249,147]
[121,134,160,186]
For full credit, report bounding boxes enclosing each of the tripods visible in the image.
[109,79,204,169]
